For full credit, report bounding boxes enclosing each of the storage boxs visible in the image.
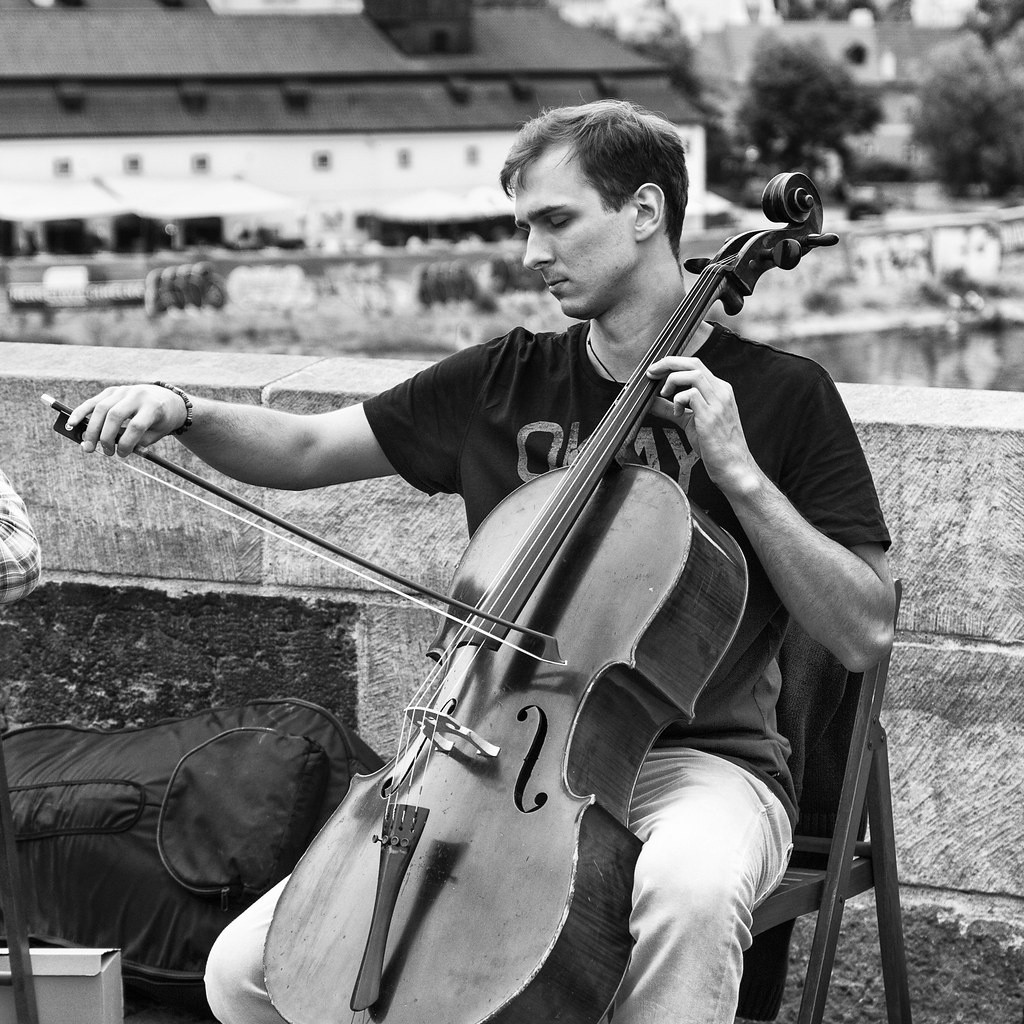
[0,947,124,1024]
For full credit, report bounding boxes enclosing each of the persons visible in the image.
[66,98,896,1023]
[0,469,41,603]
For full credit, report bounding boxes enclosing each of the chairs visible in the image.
[750,576,914,1024]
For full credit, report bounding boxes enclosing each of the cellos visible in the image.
[261,168,840,1024]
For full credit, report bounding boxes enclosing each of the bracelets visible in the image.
[155,381,192,435]
